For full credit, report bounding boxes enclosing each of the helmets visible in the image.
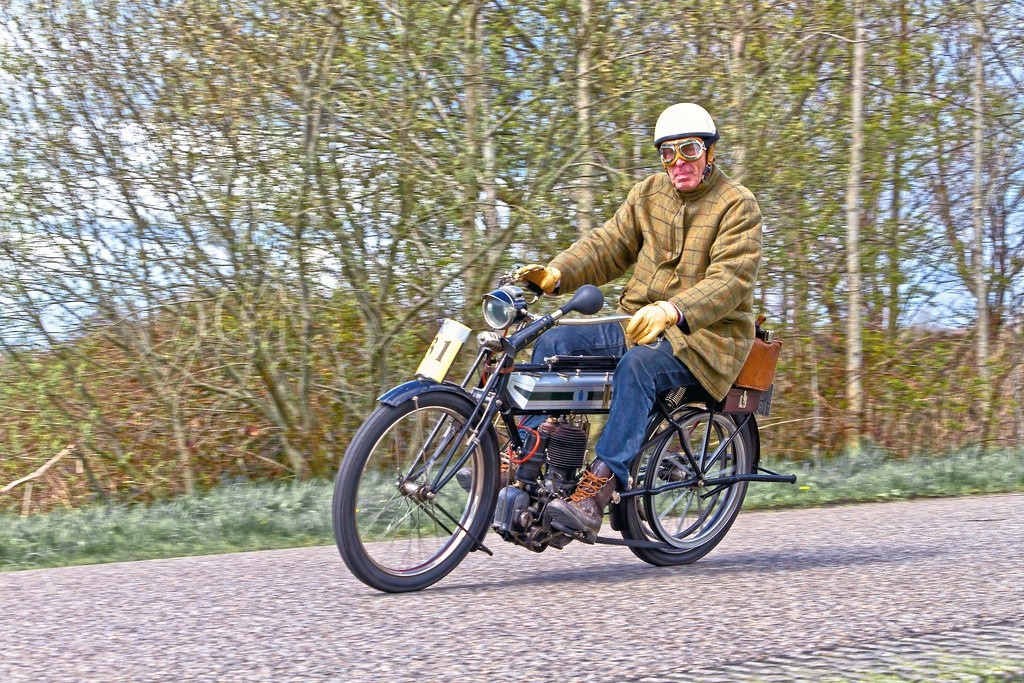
[653,102,720,148]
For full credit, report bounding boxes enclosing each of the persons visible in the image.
[457,103,763,548]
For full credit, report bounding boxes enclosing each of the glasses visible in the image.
[661,137,707,166]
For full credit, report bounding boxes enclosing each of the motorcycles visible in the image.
[329,262,796,594]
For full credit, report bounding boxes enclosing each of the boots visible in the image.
[456,444,519,494]
[547,460,617,544]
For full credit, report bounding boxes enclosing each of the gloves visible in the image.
[627,299,677,347]
[513,262,561,293]
